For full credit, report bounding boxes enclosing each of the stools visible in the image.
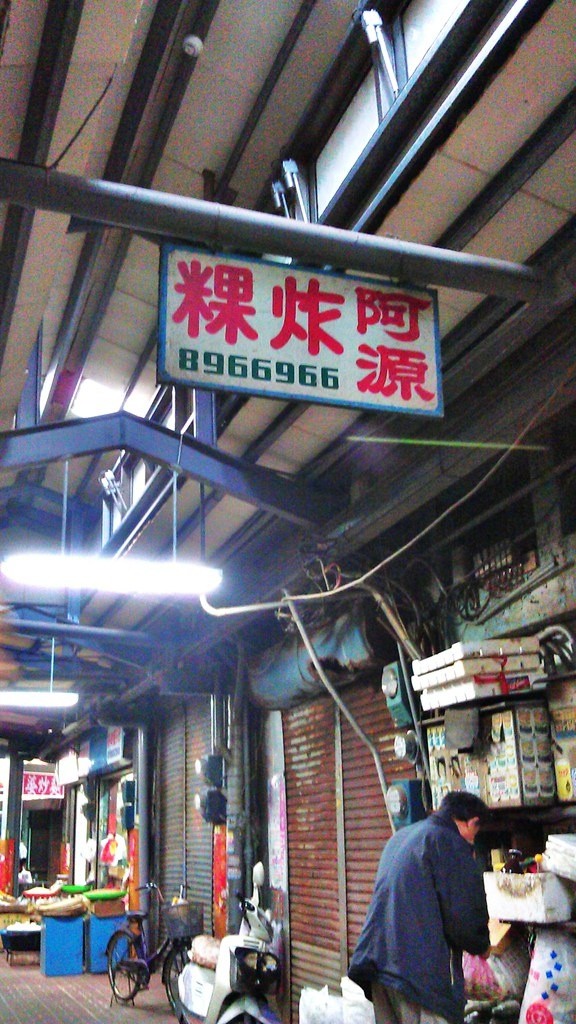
[4,950,39,965]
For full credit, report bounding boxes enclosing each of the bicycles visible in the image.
[105,882,206,1014]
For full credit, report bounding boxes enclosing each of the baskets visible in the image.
[162,902,204,938]
[230,947,281,994]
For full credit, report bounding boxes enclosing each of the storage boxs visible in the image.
[424,701,572,812]
[482,832,575,923]
[90,899,127,918]
[487,918,516,957]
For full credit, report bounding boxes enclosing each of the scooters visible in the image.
[172,861,282,1024]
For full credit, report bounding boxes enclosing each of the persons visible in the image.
[348,789,492,1024]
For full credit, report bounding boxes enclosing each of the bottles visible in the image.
[501,850,524,874]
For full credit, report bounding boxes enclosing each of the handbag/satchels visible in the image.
[461,950,500,1002]
